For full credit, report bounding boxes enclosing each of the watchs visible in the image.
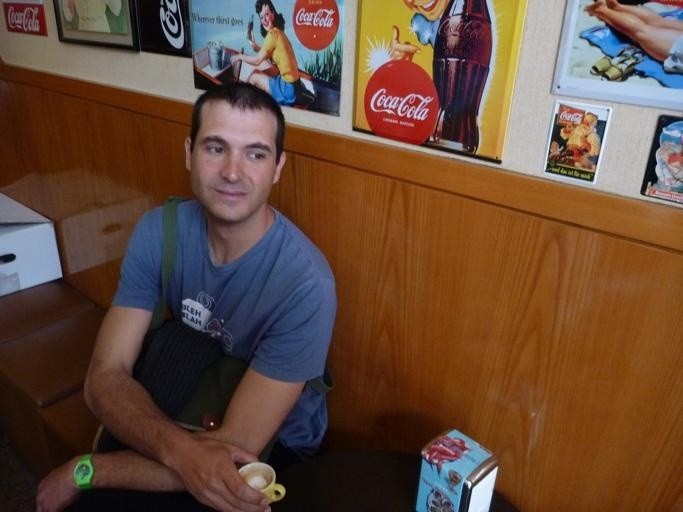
[73,454,94,491]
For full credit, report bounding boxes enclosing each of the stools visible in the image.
[0,279,111,481]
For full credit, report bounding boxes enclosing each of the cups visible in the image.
[240,461,286,505]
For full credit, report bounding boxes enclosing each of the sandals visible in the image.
[590,46,645,82]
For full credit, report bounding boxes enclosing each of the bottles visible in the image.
[427,0,493,154]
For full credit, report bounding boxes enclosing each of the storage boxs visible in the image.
[0,193,64,298]
[0,169,151,275]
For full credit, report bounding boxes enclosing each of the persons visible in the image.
[388,0,451,62]
[230,0,301,107]
[560,112,602,165]
[35,80,339,512]
[584,0,683,74]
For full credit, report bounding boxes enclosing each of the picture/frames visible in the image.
[50,0,141,54]
[548,1,682,115]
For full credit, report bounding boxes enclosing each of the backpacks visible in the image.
[92,195,252,482]
